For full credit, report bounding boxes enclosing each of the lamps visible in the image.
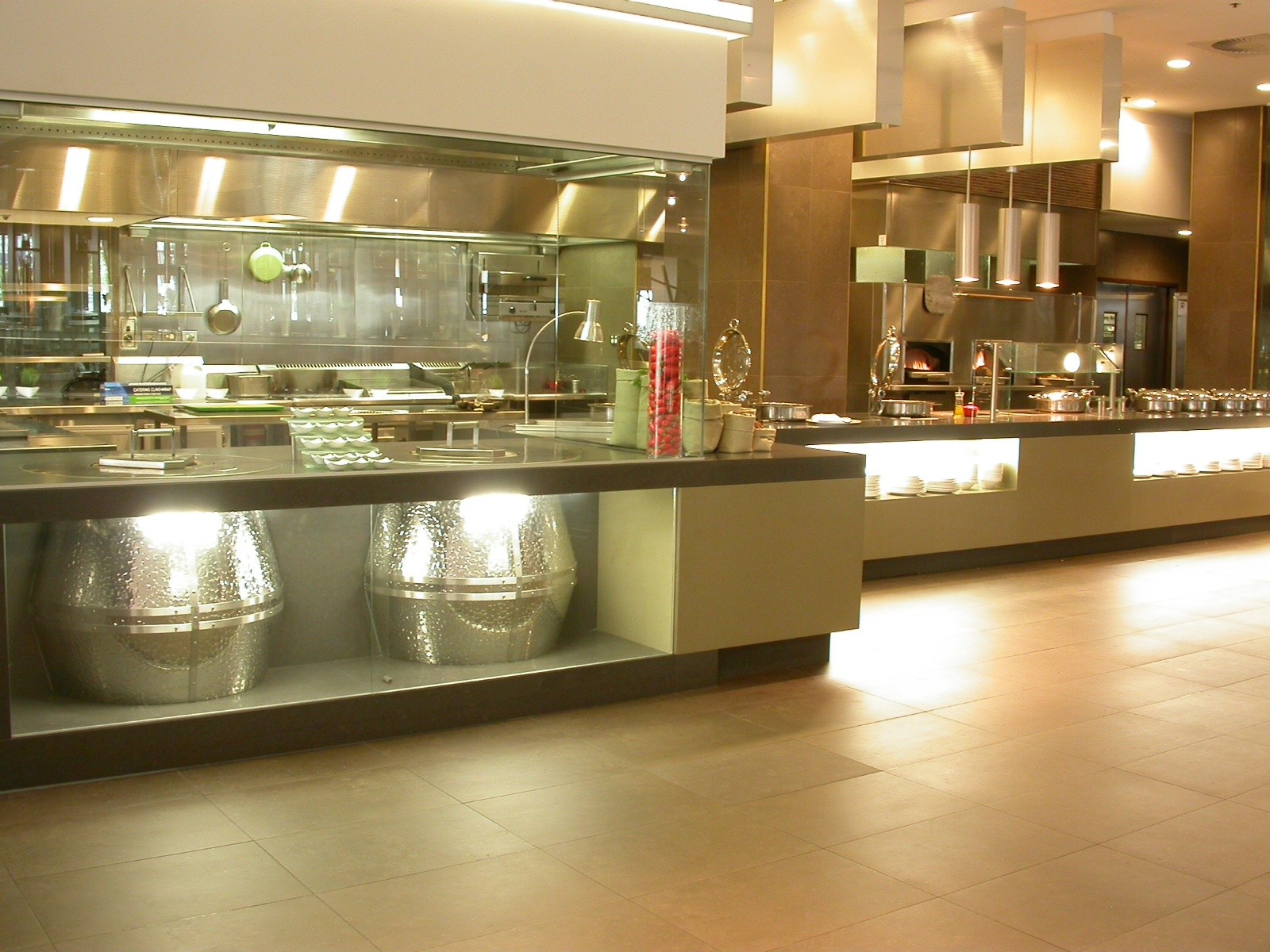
[994,168,1022,285]
[954,148,978,281]
[1035,162,1061,287]
[525,298,602,424]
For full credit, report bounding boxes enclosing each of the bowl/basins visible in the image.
[456,401,468,410]
[290,407,314,418]
[355,451,383,460]
[314,407,333,417]
[317,423,339,433]
[368,457,394,469]
[311,454,333,464]
[300,437,325,449]
[322,437,348,449]
[346,436,372,447]
[337,421,363,432]
[349,458,370,470]
[958,460,1004,490]
[372,389,389,398]
[291,422,317,434]
[324,458,349,471]
[334,453,360,461]
[334,407,355,418]
[344,389,364,398]
[588,403,615,422]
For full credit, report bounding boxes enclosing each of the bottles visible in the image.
[572,379,581,394]
[952,389,965,419]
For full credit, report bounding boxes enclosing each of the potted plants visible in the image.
[13,366,40,397]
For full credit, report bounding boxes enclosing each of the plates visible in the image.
[1133,446,1270,477]
[865,472,961,498]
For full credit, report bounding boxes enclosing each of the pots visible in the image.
[291,246,312,284]
[208,277,242,336]
[248,242,284,282]
